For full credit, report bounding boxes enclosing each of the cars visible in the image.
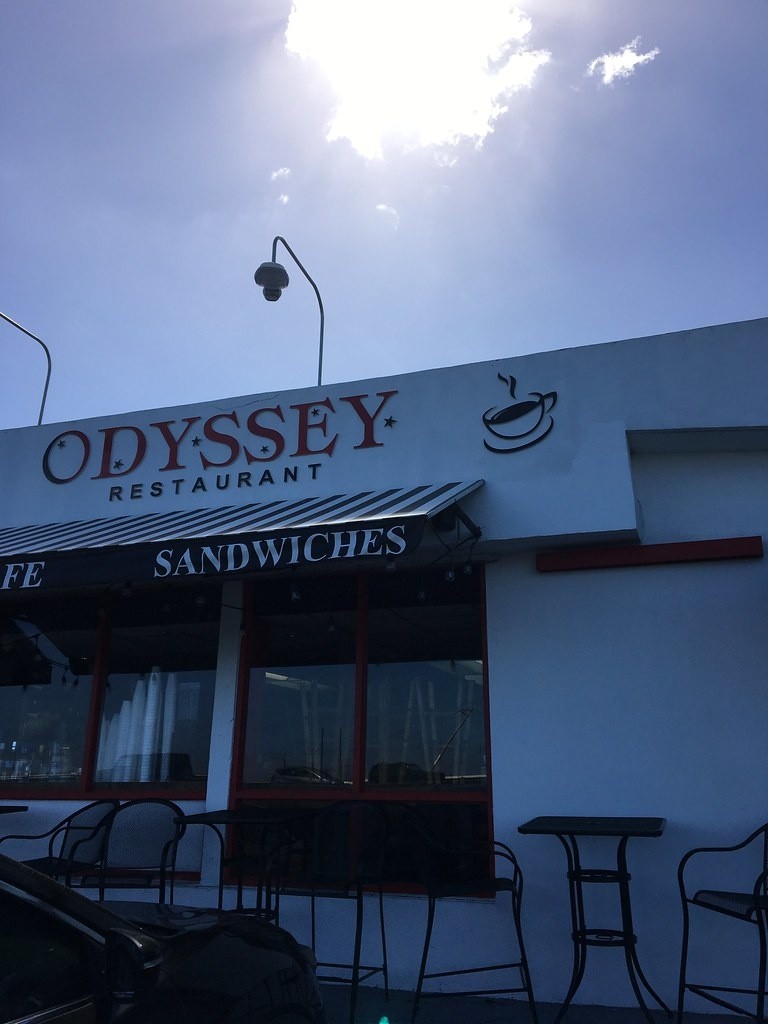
[96,753,192,782]
[270,766,344,786]
[1,852,327,1024]
[371,763,447,783]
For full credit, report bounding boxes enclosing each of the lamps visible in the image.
[254,236,325,387]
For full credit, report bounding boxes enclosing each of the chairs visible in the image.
[395,802,539,1024]
[0,797,118,902]
[677,823,768,1024]
[265,800,390,1024]
[65,796,187,903]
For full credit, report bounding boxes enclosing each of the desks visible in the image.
[517,816,674,1024]
[171,808,314,928]
[0,806,28,815]
